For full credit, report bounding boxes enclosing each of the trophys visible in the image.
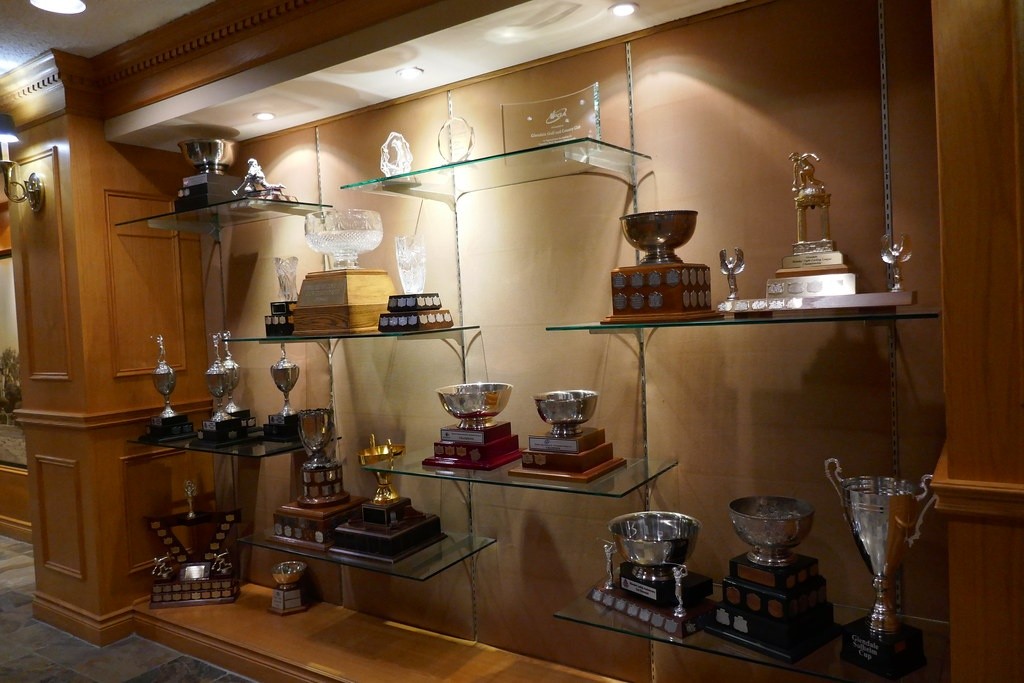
[139,118,936,679]
[500,82,601,165]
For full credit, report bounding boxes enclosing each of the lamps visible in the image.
[0,113,46,214]
